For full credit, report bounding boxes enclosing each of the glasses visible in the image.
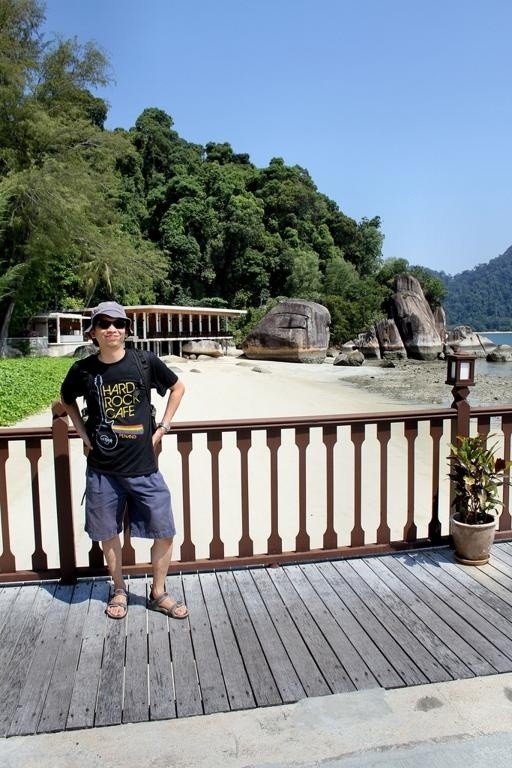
[97,319,126,329]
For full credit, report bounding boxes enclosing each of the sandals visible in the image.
[147,585,189,619]
[106,589,129,619]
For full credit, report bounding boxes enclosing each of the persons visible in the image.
[60,301,192,619]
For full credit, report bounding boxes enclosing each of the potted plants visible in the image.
[445,432,510,566]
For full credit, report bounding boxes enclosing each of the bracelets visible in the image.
[158,424,172,434]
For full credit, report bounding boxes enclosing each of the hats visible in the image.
[83,301,134,339]
